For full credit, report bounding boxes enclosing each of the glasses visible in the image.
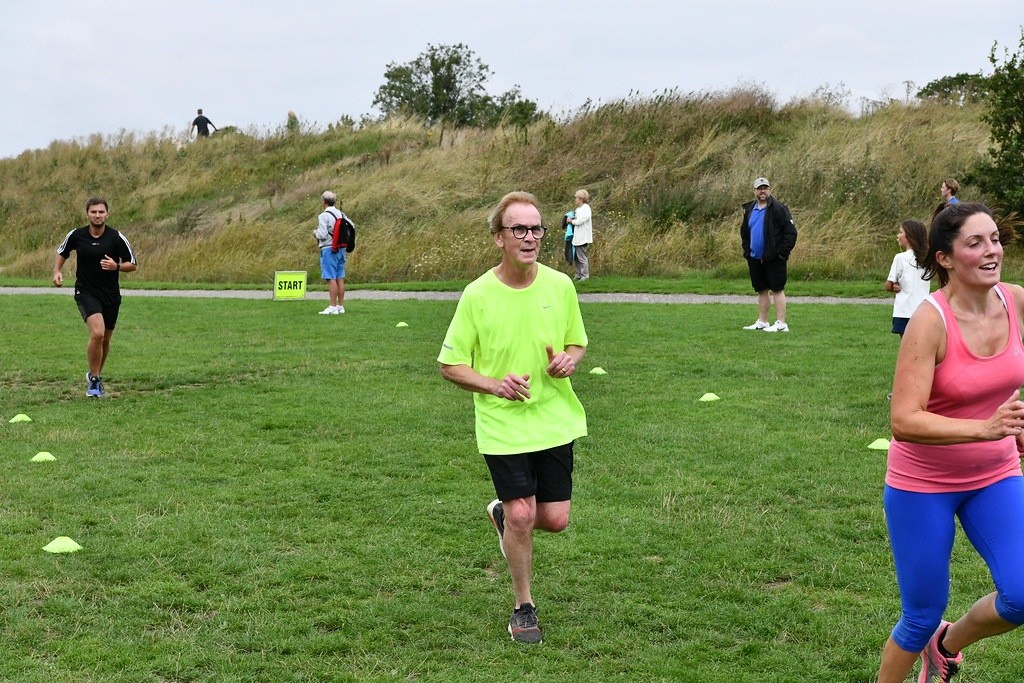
[502,226,547,239]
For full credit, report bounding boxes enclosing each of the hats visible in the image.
[321,190,336,202]
[754,178,769,189]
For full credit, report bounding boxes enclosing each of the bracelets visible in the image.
[116,264,120,271]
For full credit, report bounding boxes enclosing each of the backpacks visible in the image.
[326,211,350,253]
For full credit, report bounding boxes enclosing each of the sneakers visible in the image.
[319,307,338,315]
[508,602,543,645]
[86,370,105,398]
[918,619,962,683]
[488,498,507,559]
[336,305,346,314]
[762,320,789,331]
[743,319,770,330]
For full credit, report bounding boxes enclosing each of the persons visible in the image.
[941,178,960,204]
[312,191,355,315]
[740,177,798,332]
[874,201,1024,683]
[886,220,930,337]
[189,108,216,139]
[52,198,137,397]
[287,111,298,130]
[567,190,593,281]
[437,192,589,644]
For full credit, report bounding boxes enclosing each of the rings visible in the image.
[562,368,566,373]
